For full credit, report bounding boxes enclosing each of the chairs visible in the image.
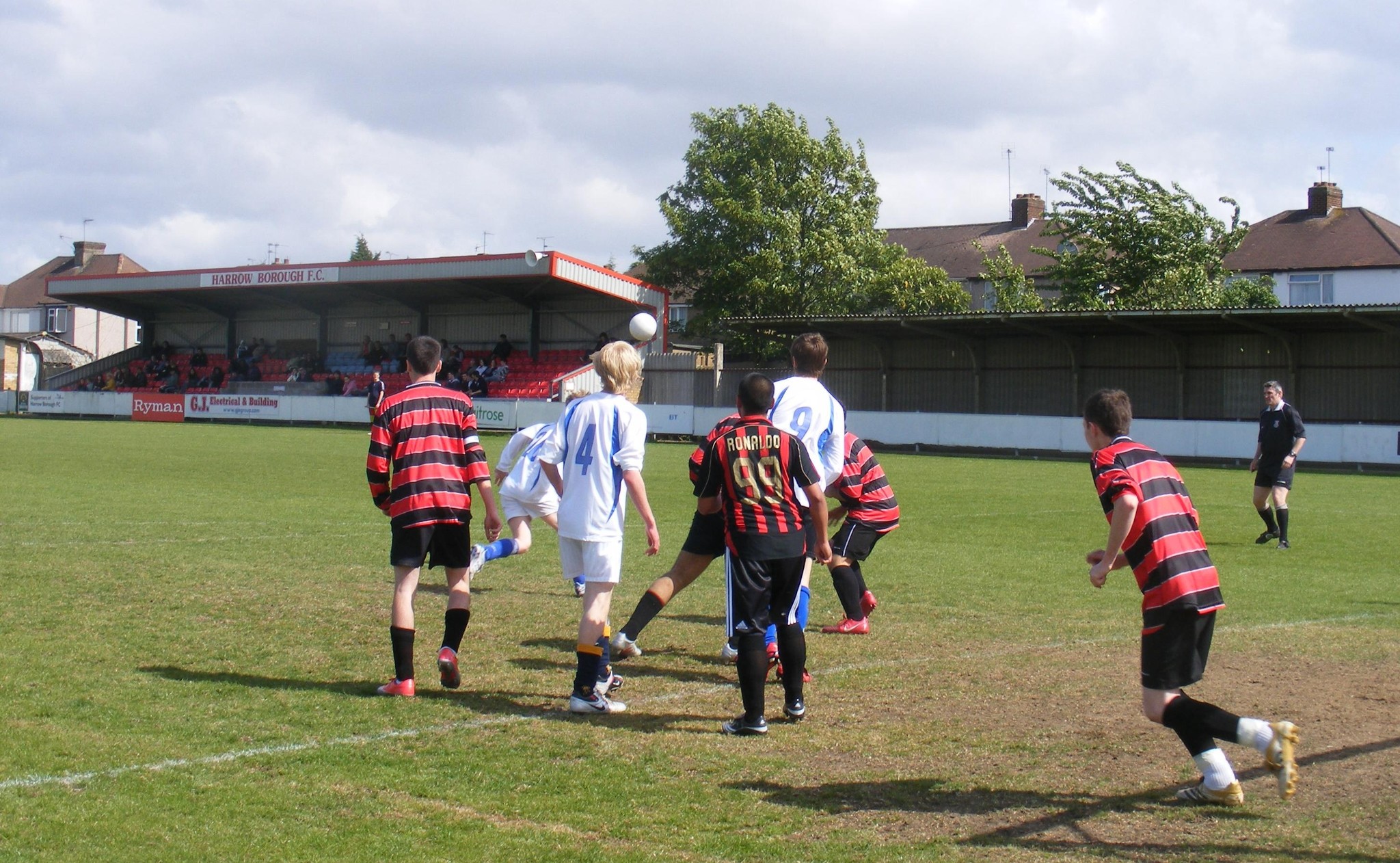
[60,349,593,401]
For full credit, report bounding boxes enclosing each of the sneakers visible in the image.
[437,646,460,688]
[377,679,414,696]
[1265,721,1301,801]
[1176,776,1244,806]
[469,544,485,582]
[765,642,779,673]
[720,644,738,665]
[594,671,624,695]
[838,590,878,626]
[822,617,869,635]
[783,699,805,722]
[570,691,626,714]
[1277,541,1288,549]
[777,663,810,682]
[575,582,586,596]
[722,713,768,735]
[612,633,641,656]
[1255,527,1280,544]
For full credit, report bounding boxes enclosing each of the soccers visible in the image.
[629,312,658,342]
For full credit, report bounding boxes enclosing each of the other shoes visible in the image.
[368,432,372,435]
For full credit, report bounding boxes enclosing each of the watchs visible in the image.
[1288,450,1297,458]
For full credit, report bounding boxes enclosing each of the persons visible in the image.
[366,371,387,435]
[609,330,901,666]
[539,339,660,715]
[1082,388,1302,809]
[75,331,610,396]
[366,335,503,698]
[470,390,592,596]
[693,372,832,736]
[1250,381,1306,548]
